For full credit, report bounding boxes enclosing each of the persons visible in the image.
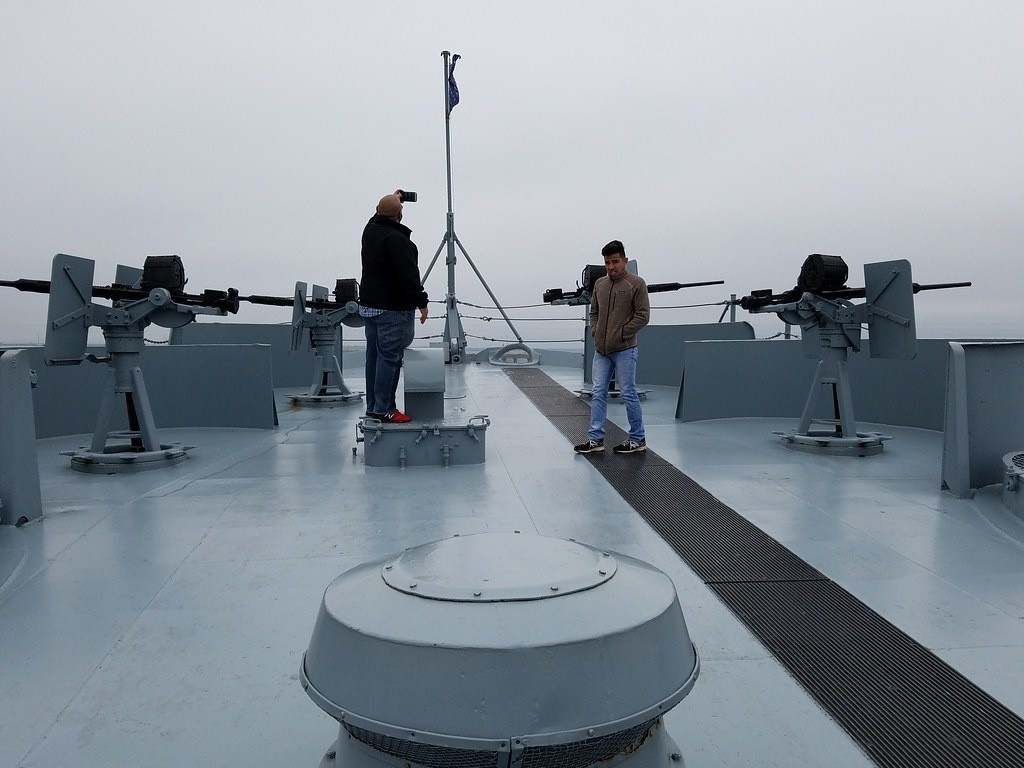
[574,240,650,452]
[359,190,430,423]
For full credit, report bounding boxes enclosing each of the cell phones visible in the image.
[401,192,417,202]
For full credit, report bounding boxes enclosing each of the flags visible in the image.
[448,54,461,116]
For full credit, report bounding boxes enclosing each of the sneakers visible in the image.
[613,437,647,452]
[574,437,605,453]
[366,408,412,423]
